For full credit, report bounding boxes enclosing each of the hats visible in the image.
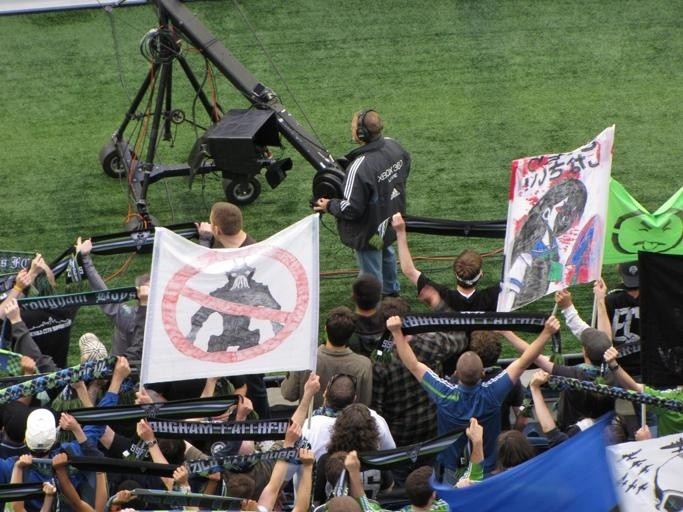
[581,327,611,363]
[618,260,640,289]
[24,408,57,454]
[2,401,30,442]
[79,332,109,365]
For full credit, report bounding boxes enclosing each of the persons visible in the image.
[0,197,682,512]
[310,104,412,300]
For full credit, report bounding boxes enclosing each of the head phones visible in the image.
[356,107,379,143]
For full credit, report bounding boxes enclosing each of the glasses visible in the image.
[328,372,358,394]
